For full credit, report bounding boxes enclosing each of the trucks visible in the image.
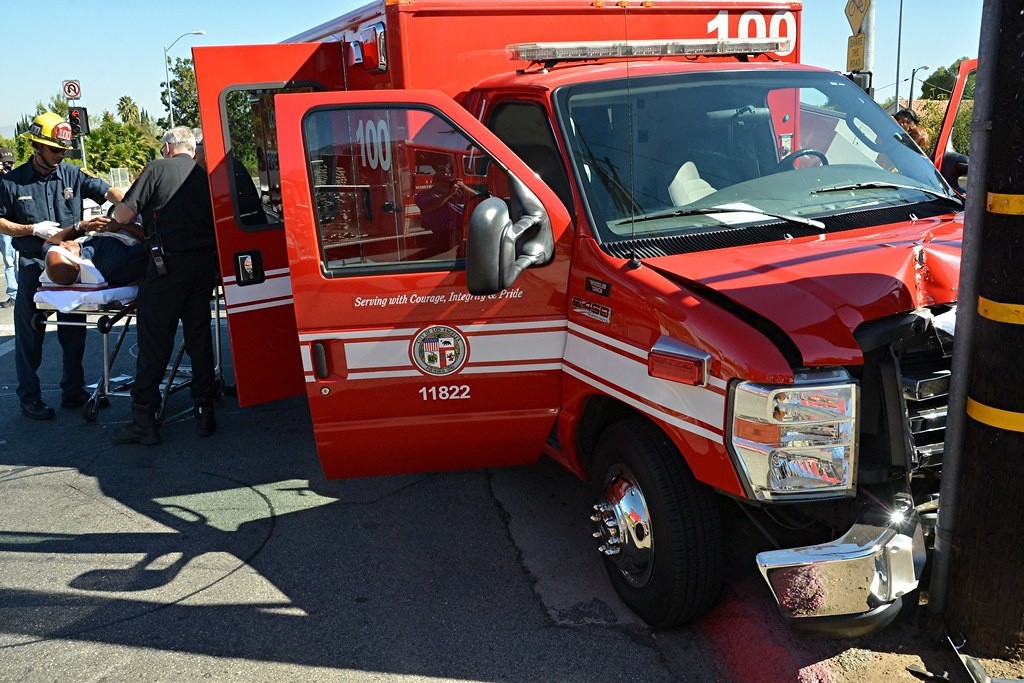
[190,0,978,640]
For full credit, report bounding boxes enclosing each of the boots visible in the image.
[193,396,217,436]
[109,403,161,445]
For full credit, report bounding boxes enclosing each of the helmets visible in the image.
[19,112,73,149]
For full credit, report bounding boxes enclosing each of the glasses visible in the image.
[48,145,67,154]
[160,142,171,157]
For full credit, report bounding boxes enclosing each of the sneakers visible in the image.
[61,389,111,408]
[19,399,56,419]
[2,298,16,308]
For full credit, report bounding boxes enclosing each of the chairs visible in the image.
[645,127,762,211]
[480,143,578,255]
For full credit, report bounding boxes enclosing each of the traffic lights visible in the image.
[67,106,88,134]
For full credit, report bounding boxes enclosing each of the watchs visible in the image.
[73,221,80,231]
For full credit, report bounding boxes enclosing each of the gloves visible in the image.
[33,221,63,240]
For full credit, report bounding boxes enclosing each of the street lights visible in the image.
[909,65,930,110]
[163,29,205,129]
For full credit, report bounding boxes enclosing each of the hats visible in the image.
[0,148,16,162]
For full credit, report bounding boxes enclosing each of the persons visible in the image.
[192,127,268,225]
[0,148,19,308]
[415,170,478,248]
[0,112,125,420]
[110,125,221,446]
[42,217,148,286]
[875,108,929,171]
